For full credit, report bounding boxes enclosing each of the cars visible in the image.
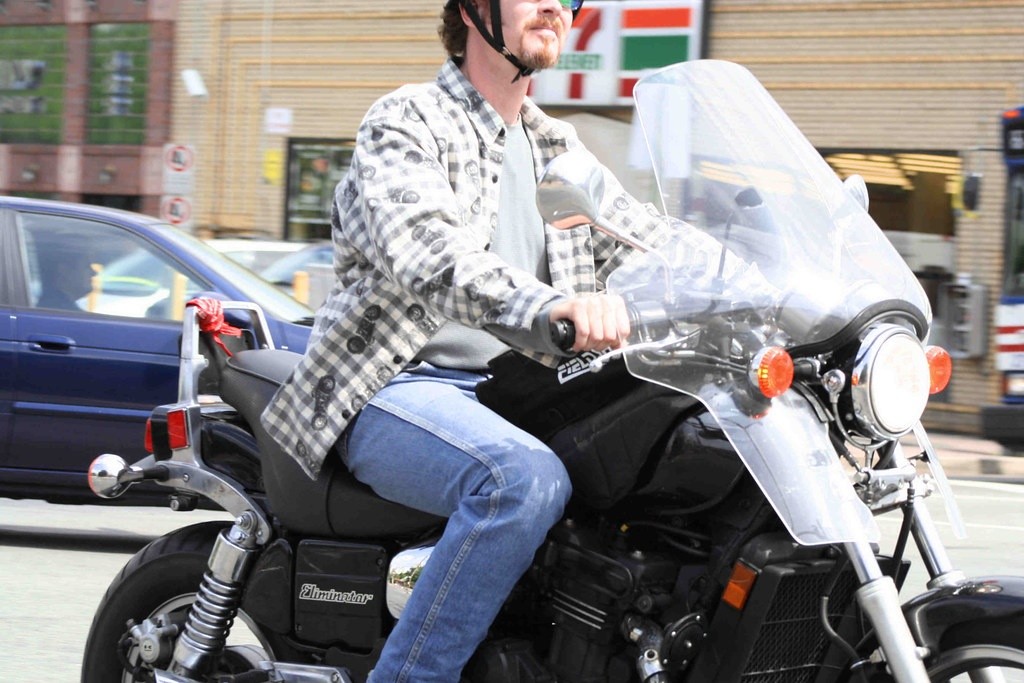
[206,237,310,275]
[0,196,317,503]
[264,242,334,305]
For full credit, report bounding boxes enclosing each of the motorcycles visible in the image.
[79,57,1022,681]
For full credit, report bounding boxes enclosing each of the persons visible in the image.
[36,234,97,313]
[260,1,776,683]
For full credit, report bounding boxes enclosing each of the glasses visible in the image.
[558,0,582,10]
